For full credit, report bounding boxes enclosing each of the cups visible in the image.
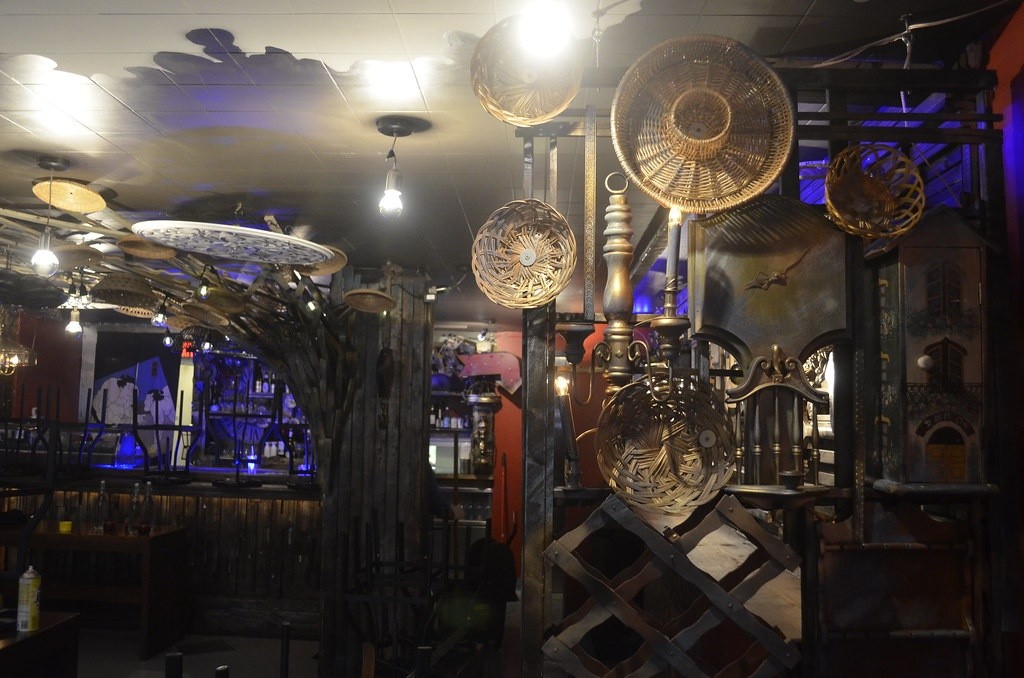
[459,458,472,474]
[58,522,74,532]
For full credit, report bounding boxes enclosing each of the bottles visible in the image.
[92,479,107,526]
[429,404,491,439]
[17,566,43,632]
[202,368,317,478]
[129,481,154,526]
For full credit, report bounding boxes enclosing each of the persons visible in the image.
[431,363,450,418]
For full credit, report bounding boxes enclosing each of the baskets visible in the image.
[471,198,577,309]
[610,34,794,214]
[470,13,584,128]
[824,145,925,239]
[48,232,397,340]
[594,372,736,514]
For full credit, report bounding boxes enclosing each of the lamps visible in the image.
[555,374,582,488]
[31,156,60,277]
[377,117,414,219]
[0,335,39,377]
[151,292,169,328]
[197,264,220,300]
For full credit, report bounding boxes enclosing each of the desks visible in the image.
[550,481,849,646]
[0,522,188,661]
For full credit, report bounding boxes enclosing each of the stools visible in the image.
[329,505,518,678]
